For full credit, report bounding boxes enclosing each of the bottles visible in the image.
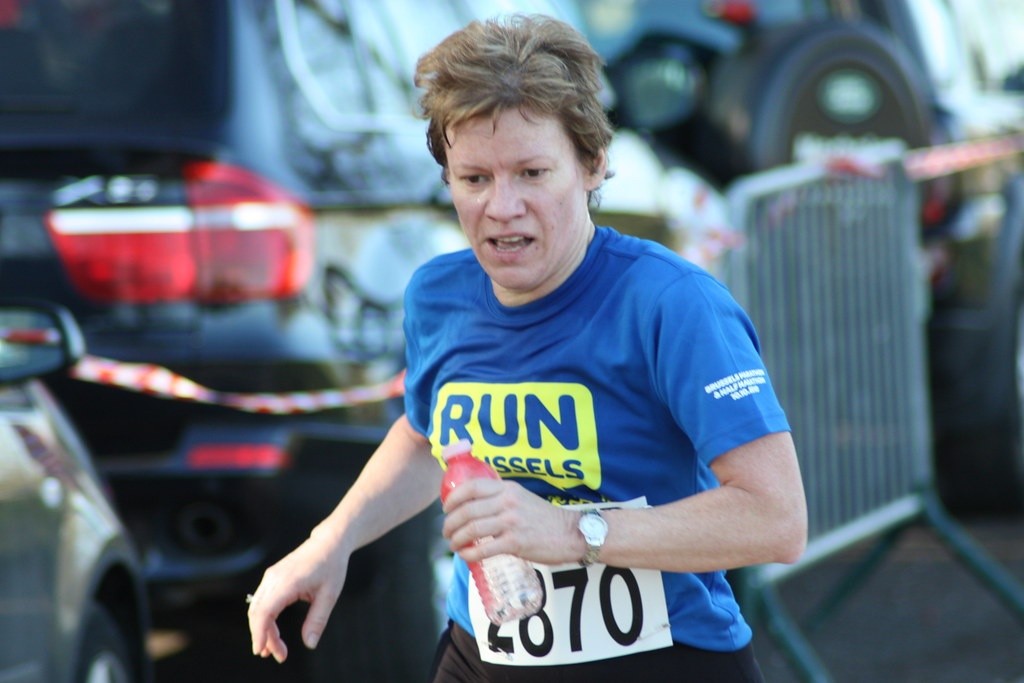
[441,439,543,626]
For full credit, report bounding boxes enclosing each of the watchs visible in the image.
[579,508,609,567]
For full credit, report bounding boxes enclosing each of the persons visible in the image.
[248,12,808,683]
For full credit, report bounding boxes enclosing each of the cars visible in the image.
[0,294,160,683]
[0,0,1024,683]
